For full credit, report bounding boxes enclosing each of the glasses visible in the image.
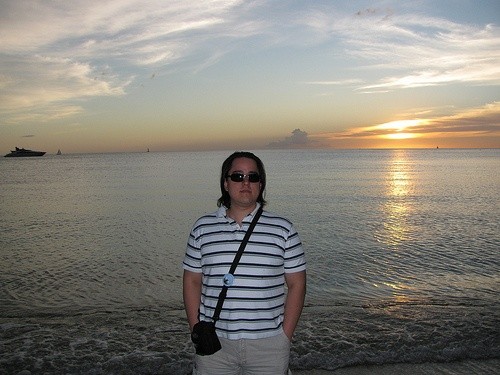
[225,173,261,183]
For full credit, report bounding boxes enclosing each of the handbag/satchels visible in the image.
[192,322,222,357]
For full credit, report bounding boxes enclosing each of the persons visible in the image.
[182,151,306,375]
